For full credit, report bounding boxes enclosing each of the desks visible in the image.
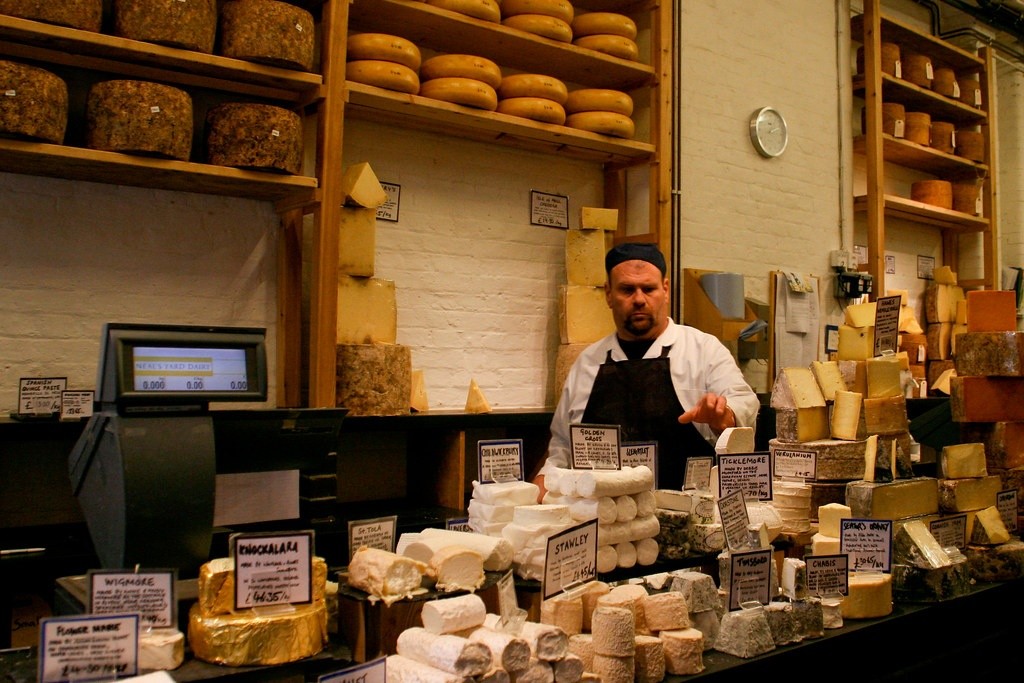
[0,548,1024,683]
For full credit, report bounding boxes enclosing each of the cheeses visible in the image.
[337,161,1024,683]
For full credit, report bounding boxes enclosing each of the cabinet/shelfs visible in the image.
[0,0,673,415]
[849,0,1000,323]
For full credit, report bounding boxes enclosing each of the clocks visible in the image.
[749,106,788,158]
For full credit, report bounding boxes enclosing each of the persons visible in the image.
[530,241,760,503]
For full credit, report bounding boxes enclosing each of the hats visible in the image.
[604,242,667,281]
